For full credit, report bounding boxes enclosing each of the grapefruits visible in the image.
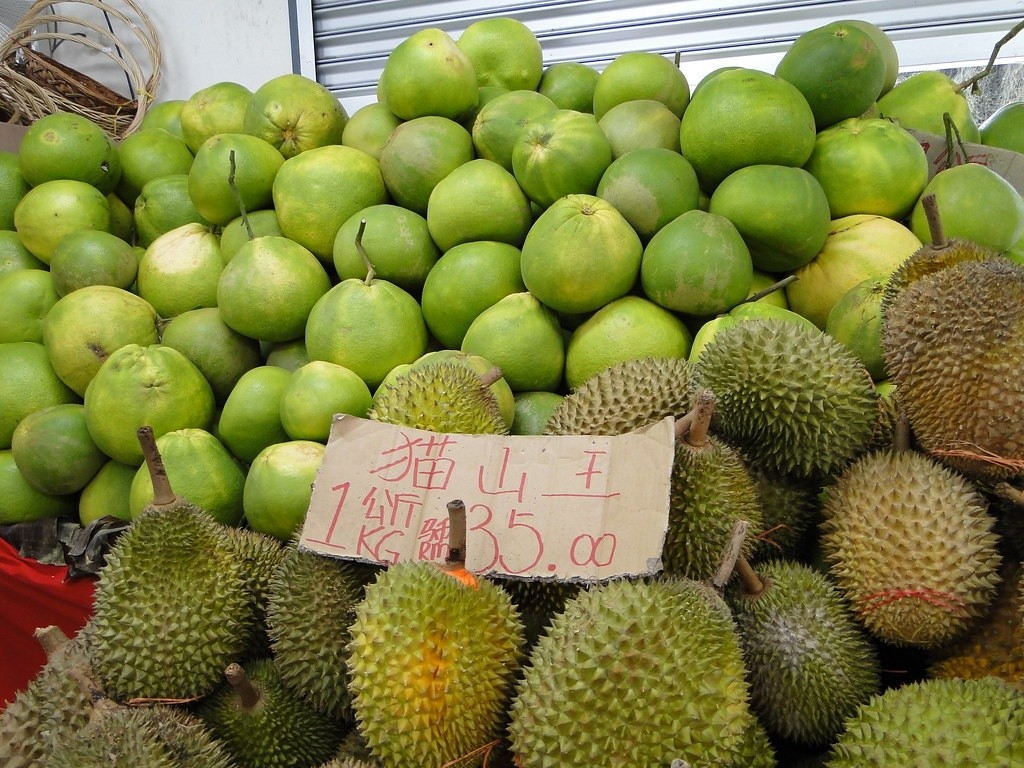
[0,16,1024,544]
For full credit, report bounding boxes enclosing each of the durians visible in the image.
[1,195,1022,768]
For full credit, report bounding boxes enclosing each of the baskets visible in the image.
[0,0,163,141]
